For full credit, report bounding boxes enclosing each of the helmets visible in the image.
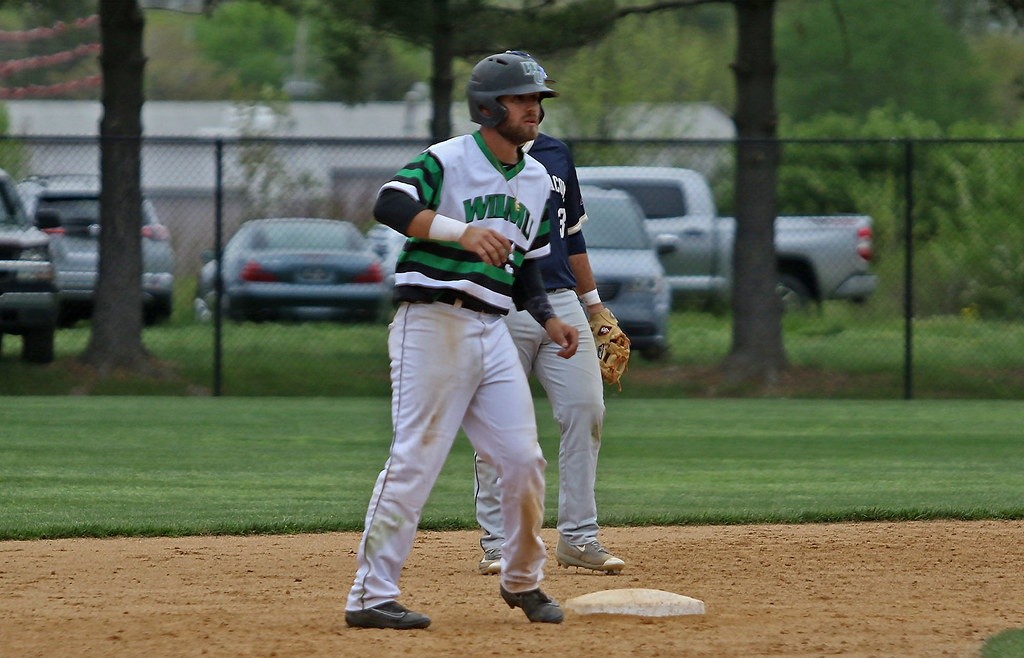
[466,54,560,129]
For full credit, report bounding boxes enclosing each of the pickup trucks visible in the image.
[368,167,877,312]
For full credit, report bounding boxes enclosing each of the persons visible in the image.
[479,51,631,575]
[345,52,564,628]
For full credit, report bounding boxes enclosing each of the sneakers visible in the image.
[344,601,431,630]
[500,580,564,624]
[478,549,503,575]
[554,533,626,571]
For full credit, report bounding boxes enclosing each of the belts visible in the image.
[435,289,509,316]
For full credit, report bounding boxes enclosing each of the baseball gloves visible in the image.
[589,308,632,393]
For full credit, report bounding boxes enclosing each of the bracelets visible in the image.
[578,287,601,308]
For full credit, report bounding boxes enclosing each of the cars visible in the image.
[0,168,63,363]
[13,173,175,326]
[580,185,681,361]
[193,218,392,326]
